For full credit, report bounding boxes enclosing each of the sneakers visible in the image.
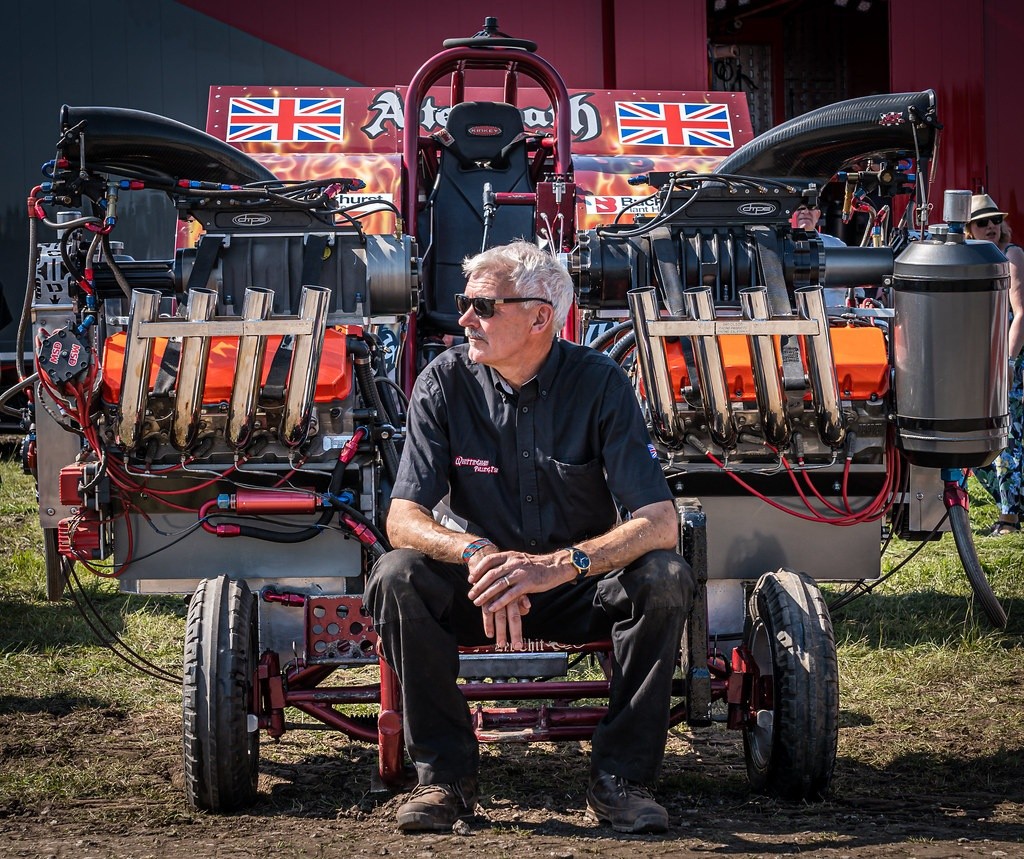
[396,780,478,831]
[585,763,669,832]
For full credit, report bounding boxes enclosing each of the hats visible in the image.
[968,194,1008,224]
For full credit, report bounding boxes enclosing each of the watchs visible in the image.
[565,546,591,585]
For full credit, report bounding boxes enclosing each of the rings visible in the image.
[504,576,510,586]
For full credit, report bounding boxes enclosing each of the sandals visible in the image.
[988,520,1022,537]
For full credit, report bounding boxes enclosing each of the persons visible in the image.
[362,236,697,832]
[792,204,865,307]
[966,193,1024,537]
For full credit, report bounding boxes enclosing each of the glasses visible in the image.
[976,214,1004,228]
[453,293,553,319]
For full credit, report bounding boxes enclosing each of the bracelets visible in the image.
[462,537,494,565]
